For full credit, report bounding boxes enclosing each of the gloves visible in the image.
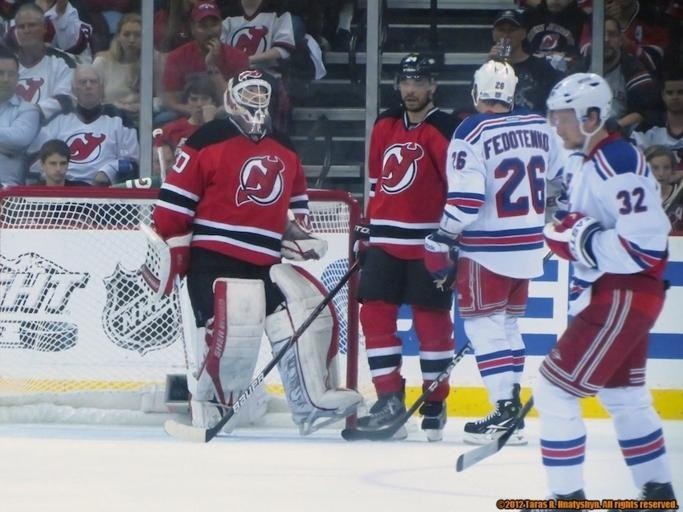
[543,210,603,271]
[426,234,458,288]
[164,231,193,297]
[353,220,366,263]
[280,209,328,260]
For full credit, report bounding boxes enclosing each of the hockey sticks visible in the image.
[455,395,534,472]
[340,249,555,442]
[163,260,361,444]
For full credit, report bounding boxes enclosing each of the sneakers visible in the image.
[357,393,407,429]
[521,490,586,512]
[608,484,678,512]
[419,402,446,430]
[465,401,524,435]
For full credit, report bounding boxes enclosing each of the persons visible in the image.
[91,12,163,120]
[154,1,202,51]
[644,145,682,237]
[669,1,683,21]
[579,0,670,71]
[308,0,356,52]
[25,64,140,188]
[25,141,94,188]
[15,3,76,127]
[354,54,462,441]
[629,68,683,170]
[468,10,565,113]
[516,73,683,512]
[514,0,541,11]
[81,0,129,54]
[520,0,592,74]
[153,1,250,126]
[154,83,218,187]
[0,45,42,188]
[425,57,578,445]
[584,15,658,138]
[6,0,92,65]
[153,67,364,437]
[219,0,295,78]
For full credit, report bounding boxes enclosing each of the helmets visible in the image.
[471,59,517,107]
[547,73,614,137]
[394,53,440,90]
[224,65,272,144]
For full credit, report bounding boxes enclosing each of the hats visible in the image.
[493,9,529,27]
[191,0,221,22]
